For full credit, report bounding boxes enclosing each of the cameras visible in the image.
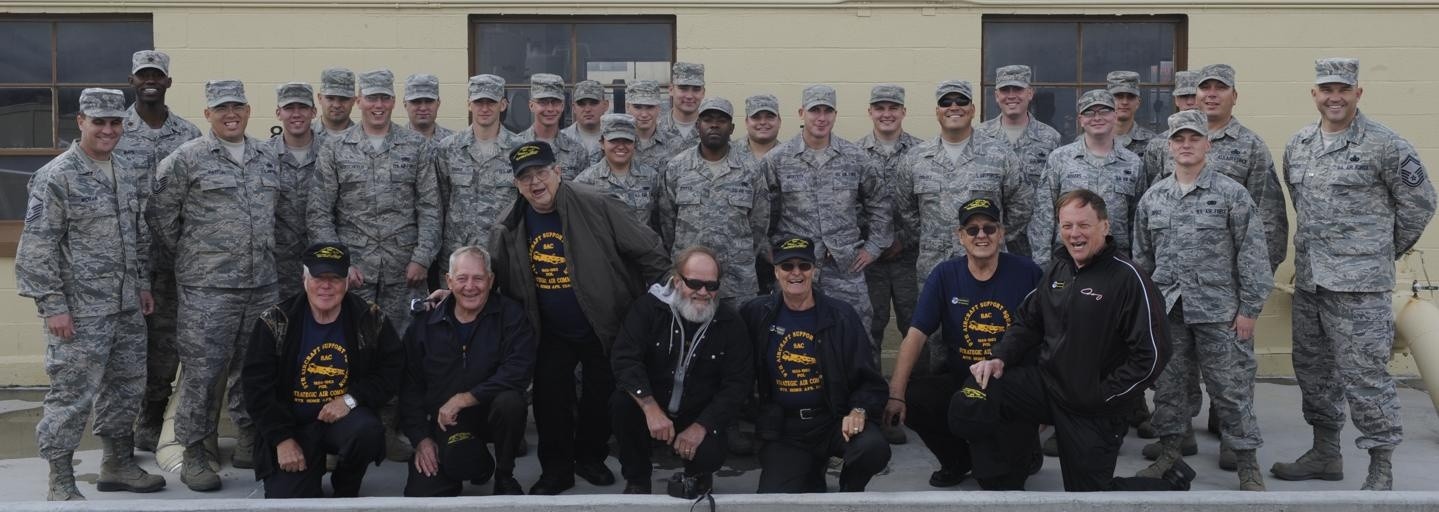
[668,471,699,499]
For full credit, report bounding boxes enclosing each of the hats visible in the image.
[773,237,814,264]
[404,74,439,102]
[994,65,1031,88]
[572,61,779,143]
[278,83,314,107]
[468,75,506,103]
[1074,63,1233,141]
[530,73,567,103]
[802,86,837,113]
[207,80,247,107]
[81,89,127,121]
[510,142,556,175]
[304,242,352,277]
[1314,59,1358,86]
[937,81,973,103]
[869,85,904,106]
[130,50,170,76]
[360,70,395,98]
[958,198,1000,224]
[319,69,356,99]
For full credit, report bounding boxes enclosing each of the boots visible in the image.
[1359,446,1394,490]
[1271,423,1345,481]
[1236,445,1266,491]
[47,449,85,501]
[179,440,222,493]
[97,433,165,492]
[1216,441,1237,471]
[230,423,260,471]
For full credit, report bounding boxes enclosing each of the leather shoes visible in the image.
[493,413,905,494]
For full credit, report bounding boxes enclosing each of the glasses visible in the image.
[780,263,812,271]
[680,272,719,292]
[964,225,997,237]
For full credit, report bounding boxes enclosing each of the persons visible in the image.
[14,49,1438,498]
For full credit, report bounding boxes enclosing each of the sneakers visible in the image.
[1131,395,1199,492]
[385,430,415,463]
[930,463,972,487]
